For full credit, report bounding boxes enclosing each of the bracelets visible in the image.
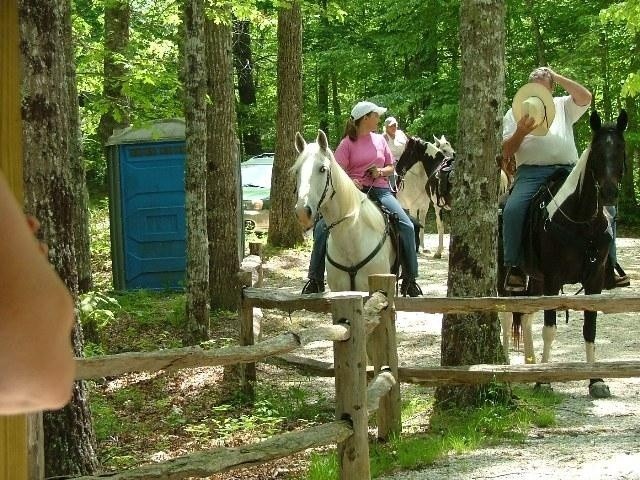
[378,168,383,177]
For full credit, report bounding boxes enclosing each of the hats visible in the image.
[512,83,556,137]
[350,101,387,120]
[385,117,397,126]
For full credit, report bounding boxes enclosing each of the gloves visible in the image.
[366,165,378,179]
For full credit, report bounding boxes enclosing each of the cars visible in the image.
[240,153,275,234]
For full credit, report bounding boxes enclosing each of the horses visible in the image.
[395,133,507,258]
[288,129,402,293]
[497,109,628,398]
[395,134,456,255]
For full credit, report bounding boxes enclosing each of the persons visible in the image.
[501,66,630,292]
[301,100,423,299]
[1,173,77,416]
[381,117,408,190]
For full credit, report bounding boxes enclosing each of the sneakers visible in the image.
[400,278,422,297]
[615,275,630,284]
[303,278,326,295]
[507,274,525,287]
